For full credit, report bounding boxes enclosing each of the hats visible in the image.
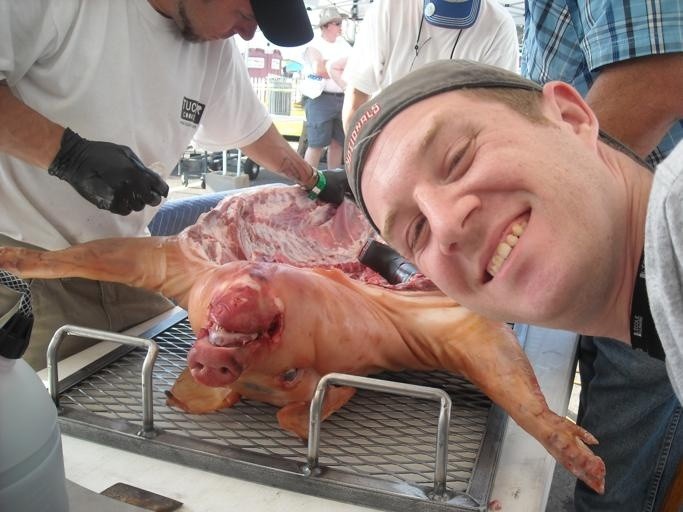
[249,0,313,46]
[423,0,481,27]
[314,7,347,27]
[344,58,652,235]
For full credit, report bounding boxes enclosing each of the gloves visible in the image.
[48,128,170,216]
[314,169,352,206]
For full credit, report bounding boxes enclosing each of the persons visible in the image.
[342,2,519,136]
[342,58,683,402]
[0,0,353,371]
[522,1,683,512]
[303,5,355,172]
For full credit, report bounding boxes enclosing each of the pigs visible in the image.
[0,185,606,495]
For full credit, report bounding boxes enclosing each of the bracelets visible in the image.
[300,165,317,190]
[307,167,326,200]
